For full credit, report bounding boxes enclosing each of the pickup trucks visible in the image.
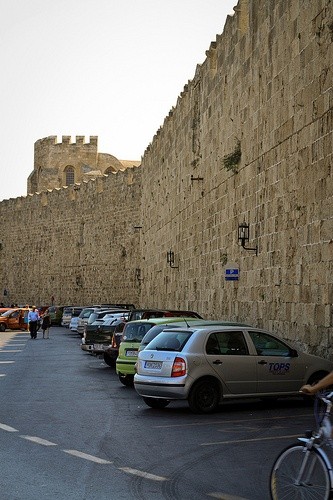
[81,309,206,365]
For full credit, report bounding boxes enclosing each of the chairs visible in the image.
[227,335,248,355]
[208,339,220,354]
[166,338,180,350]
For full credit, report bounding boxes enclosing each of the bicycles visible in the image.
[268,388,333,500]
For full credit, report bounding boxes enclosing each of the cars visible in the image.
[0,308,13,316]
[0,309,40,332]
[138,321,254,355]
[133,325,332,412]
[82,310,131,343]
[2,303,136,337]
[115,315,209,388]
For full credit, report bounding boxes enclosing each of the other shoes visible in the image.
[34,337,36,339]
[31,337,33,339]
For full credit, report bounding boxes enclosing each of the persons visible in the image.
[28,308,39,339]
[42,309,51,339]
[300,371,333,395]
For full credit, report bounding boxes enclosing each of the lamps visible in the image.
[166,250,179,271]
[238,218,257,256]
